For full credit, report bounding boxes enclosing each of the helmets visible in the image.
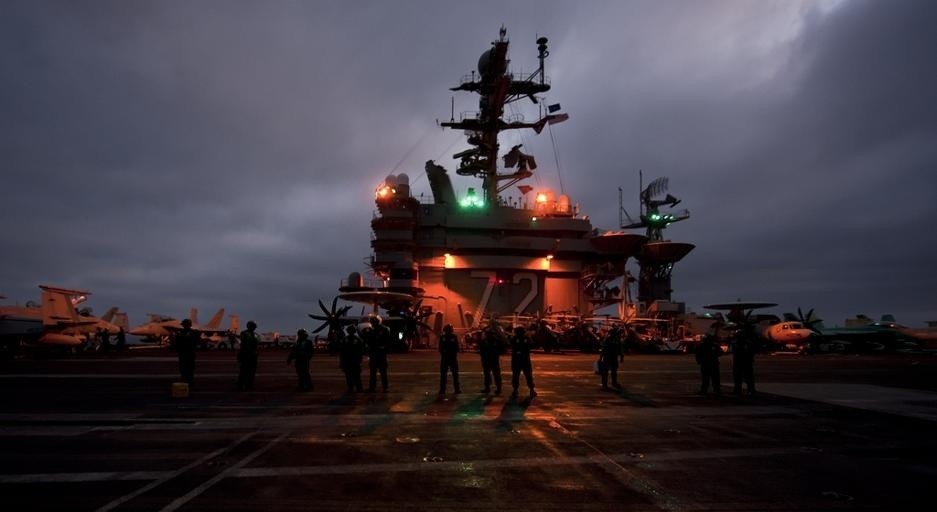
[368,315,382,326]
[298,329,308,336]
[181,319,192,325]
[246,321,257,328]
[515,326,523,333]
[443,324,452,331]
[346,326,355,333]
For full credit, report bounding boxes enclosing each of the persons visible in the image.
[169,319,203,385]
[508,324,538,397]
[365,315,392,391]
[729,323,757,397]
[597,325,624,389]
[337,326,366,391]
[236,319,262,388]
[286,327,315,390]
[695,322,721,396]
[474,319,506,395]
[112,325,126,347]
[438,323,460,395]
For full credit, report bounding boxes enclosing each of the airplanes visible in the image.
[697,298,937,356]
[308,295,439,346]
[0,284,240,350]
